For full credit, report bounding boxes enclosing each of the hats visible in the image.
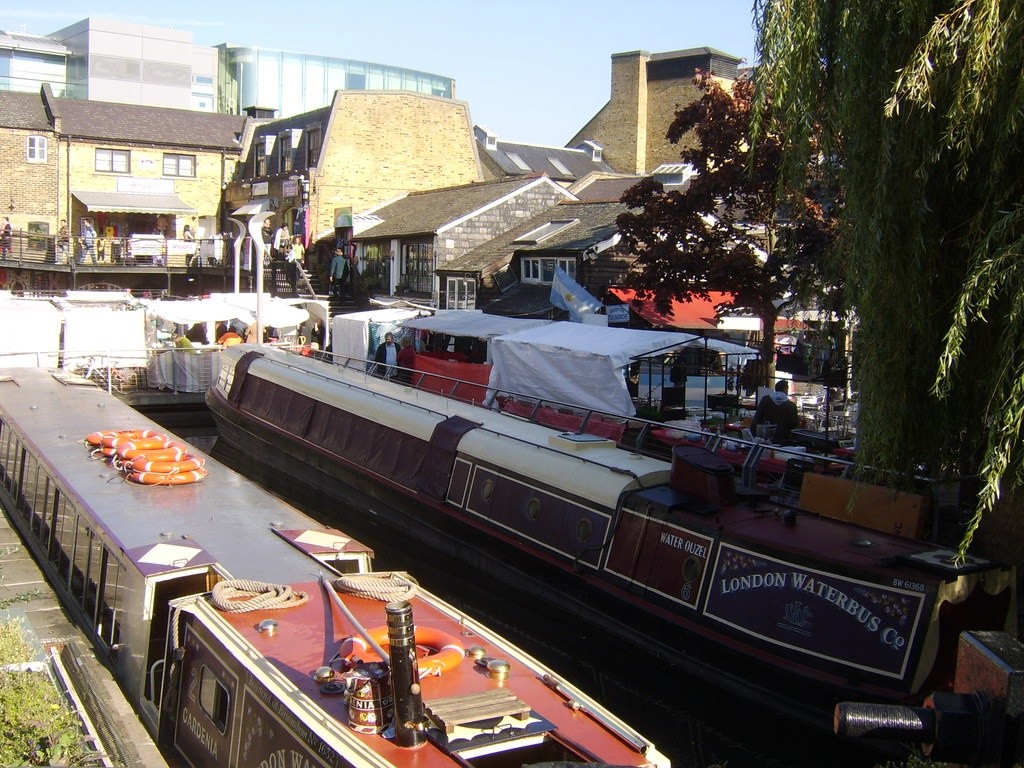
[335,249,343,256]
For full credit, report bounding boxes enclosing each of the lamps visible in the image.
[462,272,472,286]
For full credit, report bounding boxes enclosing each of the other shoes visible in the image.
[76,261,85,264]
[55,262,62,265]
[92,263,97,265]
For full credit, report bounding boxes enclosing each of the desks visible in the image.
[652,429,846,482]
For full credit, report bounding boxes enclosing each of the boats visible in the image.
[205,351,1011,748]
[403,312,968,541]
[1,367,674,768]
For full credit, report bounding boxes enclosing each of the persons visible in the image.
[311,318,324,350]
[330,249,349,304]
[150,224,195,268]
[233,319,267,344]
[374,332,413,384]
[171,334,195,354]
[0,217,12,259]
[77,219,98,265]
[263,219,305,291]
[54,220,73,265]
[749,380,799,446]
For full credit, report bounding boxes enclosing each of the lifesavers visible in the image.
[101,441,205,472]
[130,467,208,486]
[85,428,168,450]
[339,625,464,680]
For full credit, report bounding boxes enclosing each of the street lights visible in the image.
[57,139,85,261]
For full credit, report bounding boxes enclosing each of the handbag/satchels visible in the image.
[342,259,349,279]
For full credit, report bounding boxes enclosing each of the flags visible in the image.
[548,263,603,322]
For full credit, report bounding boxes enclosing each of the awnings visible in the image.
[71,191,198,215]
[230,198,271,215]
[608,288,817,331]
